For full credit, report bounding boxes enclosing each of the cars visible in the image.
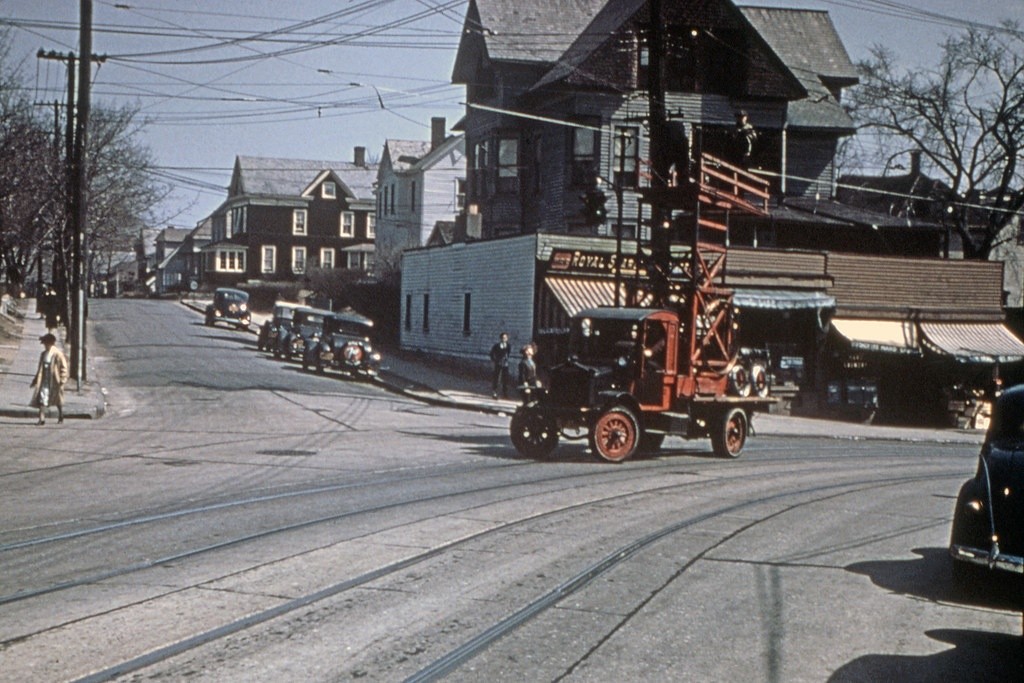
[257,303,312,351]
[303,313,381,381]
[205,288,251,330]
[949,385,1024,574]
[273,306,336,361]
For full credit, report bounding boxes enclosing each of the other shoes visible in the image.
[493,393,499,400]
[57,417,64,423]
[34,420,45,425]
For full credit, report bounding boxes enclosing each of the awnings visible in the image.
[831,319,923,356]
[544,276,654,330]
[919,323,1024,365]
[733,289,835,309]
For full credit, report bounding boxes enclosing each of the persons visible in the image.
[727,108,756,166]
[518,345,537,402]
[489,333,512,400]
[43,283,58,334]
[36,279,46,319]
[28,334,67,425]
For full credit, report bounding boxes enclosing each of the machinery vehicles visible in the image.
[510,152,780,463]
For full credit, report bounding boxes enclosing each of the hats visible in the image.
[39,333,56,343]
